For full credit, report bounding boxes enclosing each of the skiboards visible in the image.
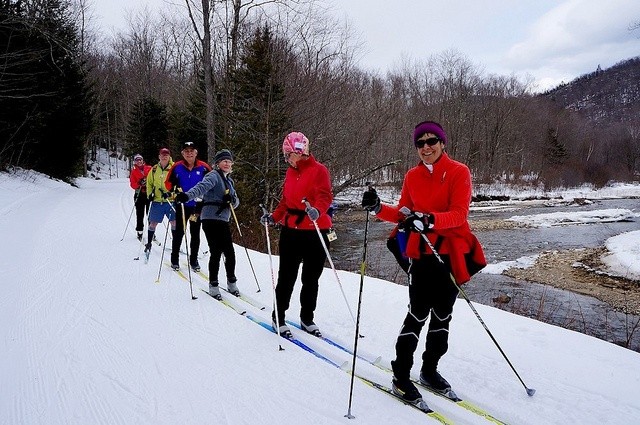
[163,253,209,282]
[143,243,204,264]
[134,226,162,248]
[347,363,508,425]
[243,309,382,370]
[196,278,265,314]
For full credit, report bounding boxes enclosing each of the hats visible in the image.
[159,147,170,153]
[182,141,196,149]
[414,120,449,145]
[214,148,233,164]
[283,131,310,155]
[134,154,143,160]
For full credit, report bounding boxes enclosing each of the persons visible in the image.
[167,138,211,273]
[144,147,175,268]
[362,120,488,415]
[261,131,332,340]
[177,148,241,299]
[131,154,154,241]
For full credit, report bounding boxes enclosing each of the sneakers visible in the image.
[272,320,290,334]
[392,376,422,403]
[227,276,239,293]
[153,235,156,240]
[301,320,319,333]
[190,259,200,269]
[209,280,221,297]
[171,261,179,268]
[420,369,451,393]
[137,230,143,236]
[144,242,152,252]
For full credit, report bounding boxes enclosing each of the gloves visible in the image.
[161,191,171,200]
[176,191,189,202]
[259,212,275,226]
[305,206,320,220]
[149,192,154,200]
[361,187,382,215]
[406,210,434,232]
[223,194,236,204]
[140,179,145,186]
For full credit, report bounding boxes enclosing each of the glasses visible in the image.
[415,135,440,148]
[283,151,293,158]
